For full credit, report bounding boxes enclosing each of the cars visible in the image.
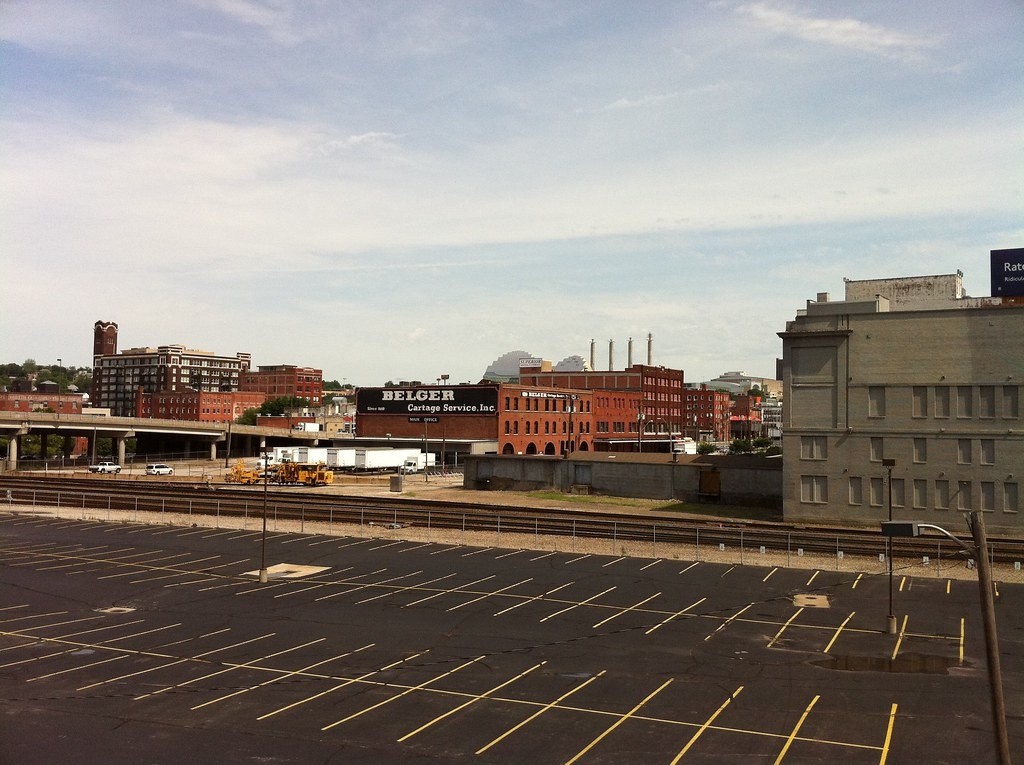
[89,461,122,474]
[145,464,173,476]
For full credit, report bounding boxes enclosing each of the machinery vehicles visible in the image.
[224,457,333,487]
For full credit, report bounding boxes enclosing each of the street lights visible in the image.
[57,359,61,419]
[639,420,653,452]
[880,509,1011,765]
[657,417,672,453]
[259,445,274,583]
[881,457,897,635]
